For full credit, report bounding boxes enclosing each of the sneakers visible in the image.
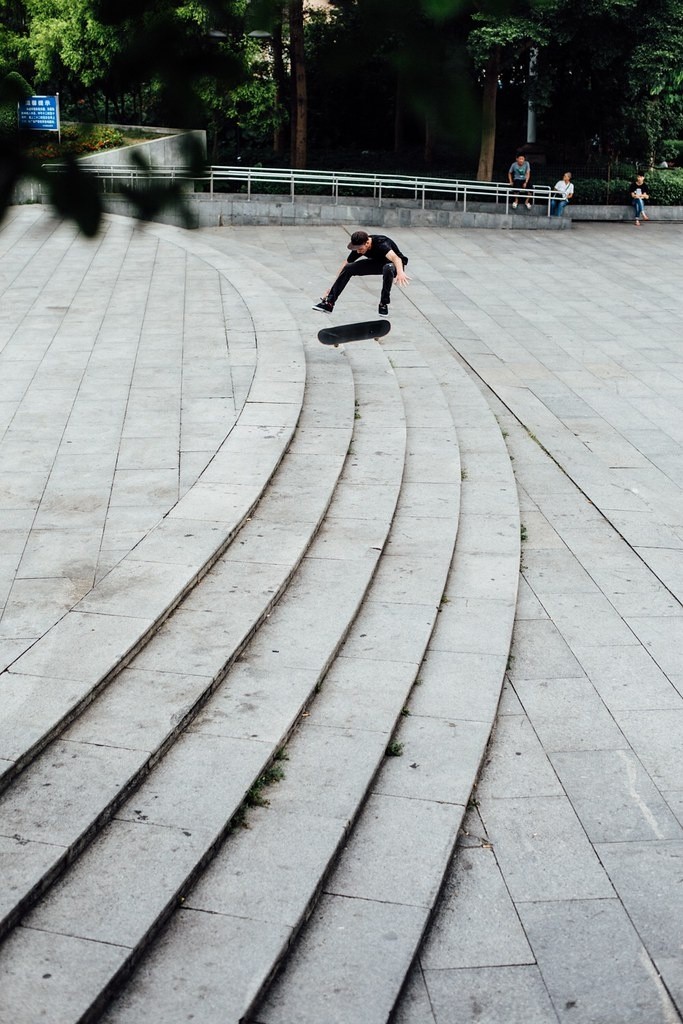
[378,303,388,316]
[312,297,333,314]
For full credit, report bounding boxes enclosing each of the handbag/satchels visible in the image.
[554,193,562,203]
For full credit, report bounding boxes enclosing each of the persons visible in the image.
[629,170,650,227]
[550,172,575,217]
[507,153,534,208]
[312,231,412,317]
[658,157,668,168]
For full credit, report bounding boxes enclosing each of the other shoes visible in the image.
[525,202,531,209]
[512,201,517,209]
[636,220,640,226]
[642,213,648,220]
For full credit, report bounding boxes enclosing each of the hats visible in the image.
[635,170,645,177]
[565,172,571,178]
[347,231,368,250]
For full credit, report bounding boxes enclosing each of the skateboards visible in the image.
[317,319,392,348]
